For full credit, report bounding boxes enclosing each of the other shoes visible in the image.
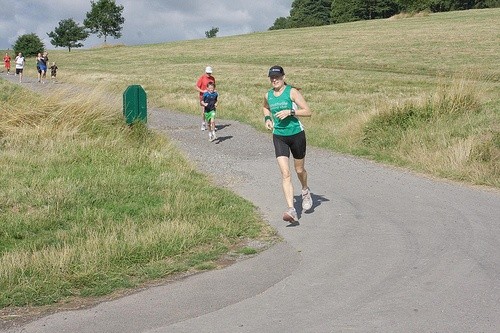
[201,124,206,131]
[209,138,219,144]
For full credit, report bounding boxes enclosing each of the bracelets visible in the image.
[264,116,272,121]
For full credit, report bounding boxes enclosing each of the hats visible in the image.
[268,66,284,77]
[205,66,212,74]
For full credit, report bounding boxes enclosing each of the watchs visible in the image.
[290,109,295,116]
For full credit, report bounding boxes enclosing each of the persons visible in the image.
[261,65,312,225]
[200,82,219,144]
[195,67,218,131]
[3,52,12,75]
[35,51,58,84]
[13,53,25,83]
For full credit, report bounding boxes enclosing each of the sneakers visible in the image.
[302,186,313,209]
[283,209,299,225]
